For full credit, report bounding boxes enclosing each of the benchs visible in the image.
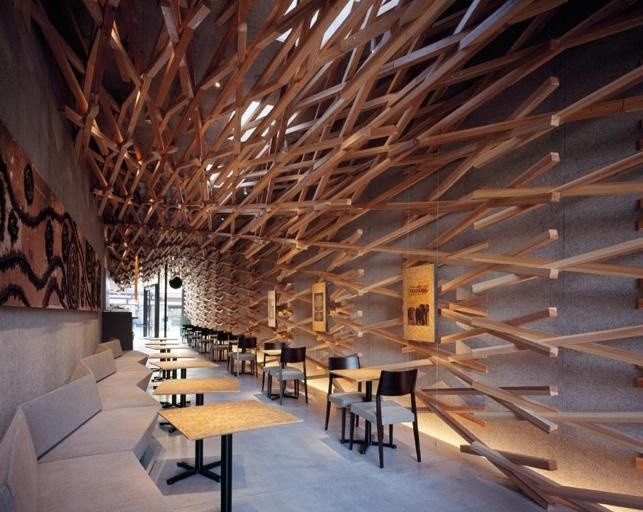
[0,338,174,512]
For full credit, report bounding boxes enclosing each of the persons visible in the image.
[408,304,429,326]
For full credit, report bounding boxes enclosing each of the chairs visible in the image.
[182,324,421,469]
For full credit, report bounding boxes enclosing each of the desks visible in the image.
[144,338,304,512]
